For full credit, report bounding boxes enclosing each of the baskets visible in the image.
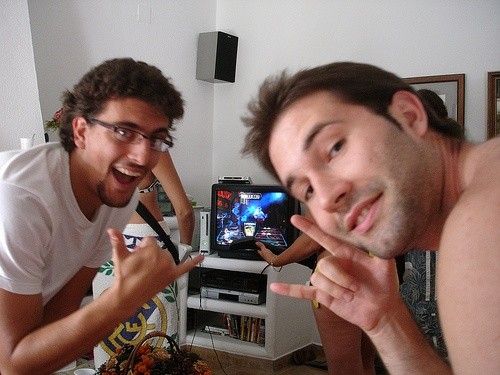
[95,331,211,375]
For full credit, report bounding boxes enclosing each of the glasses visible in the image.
[85,118,174,153]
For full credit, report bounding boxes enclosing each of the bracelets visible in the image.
[271,255,282,273]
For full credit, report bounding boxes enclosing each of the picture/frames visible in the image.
[401,74,465,139]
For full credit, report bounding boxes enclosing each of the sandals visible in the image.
[293,346,317,364]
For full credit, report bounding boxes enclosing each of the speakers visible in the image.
[195,31,238,83]
[200,209,212,256]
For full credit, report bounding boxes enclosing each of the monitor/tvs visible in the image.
[210,184,302,262]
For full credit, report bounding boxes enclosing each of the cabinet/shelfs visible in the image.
[177,254,312,361]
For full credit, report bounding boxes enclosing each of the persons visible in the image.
[240,60,500,374]
[0,56,195,375]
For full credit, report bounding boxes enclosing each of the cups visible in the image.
[21,138,34,150]
[244,222,256,236]
[74,368,96,375]
